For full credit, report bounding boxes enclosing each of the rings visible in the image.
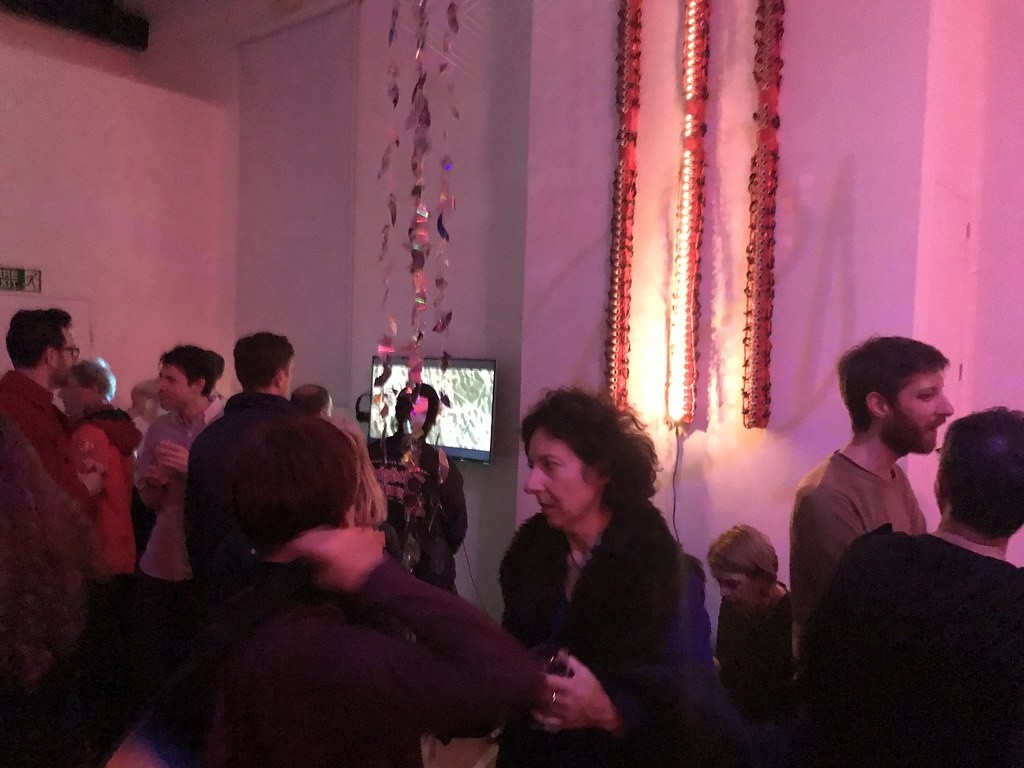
[552,691,556,704]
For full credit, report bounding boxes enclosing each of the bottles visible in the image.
[529,647,576,725]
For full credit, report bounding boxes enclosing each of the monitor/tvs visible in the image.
[367,356,496,465]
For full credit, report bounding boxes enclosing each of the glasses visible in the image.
[39,344,80,362]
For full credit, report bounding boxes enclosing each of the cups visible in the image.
[130,440,158,474]
[67,441,95,472]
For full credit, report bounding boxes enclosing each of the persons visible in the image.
[1,305,1024,768]
